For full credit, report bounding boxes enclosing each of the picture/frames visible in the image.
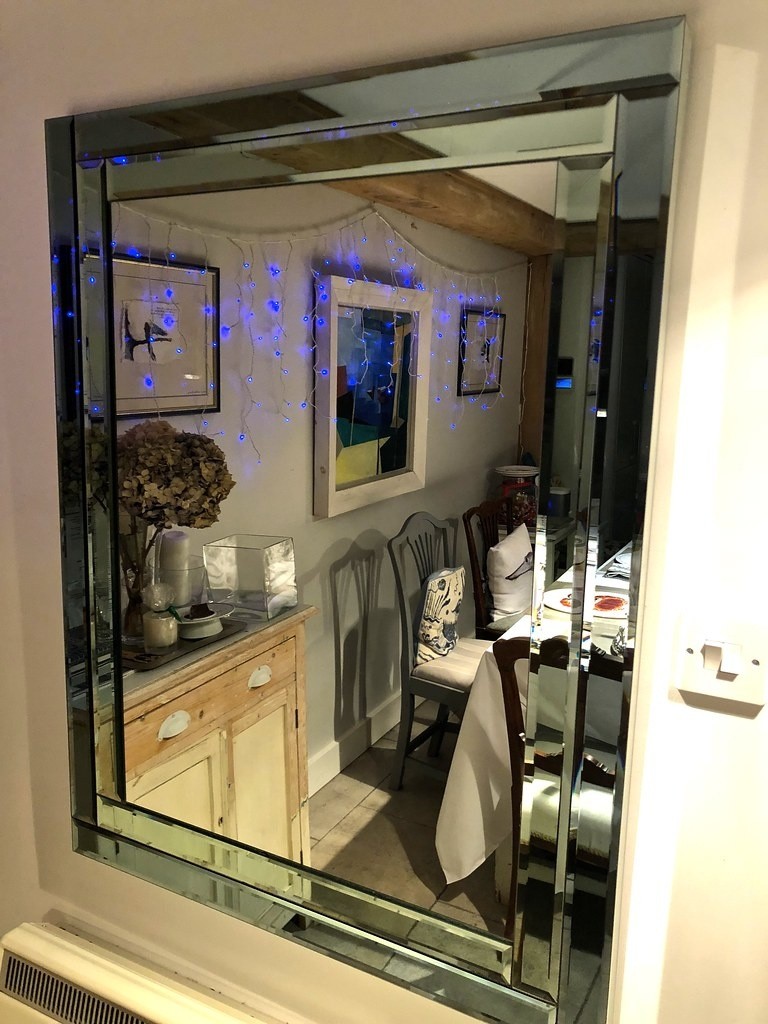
[456,308,506,395]
[314,275,434,517]
[79,250,222,422]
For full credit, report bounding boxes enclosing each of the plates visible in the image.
[616,553,632,565]
[542,588,630,618]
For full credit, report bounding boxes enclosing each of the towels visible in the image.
[604,560,632,579]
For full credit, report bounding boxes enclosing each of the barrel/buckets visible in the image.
[547,487,570,519]
[494,466,541,525]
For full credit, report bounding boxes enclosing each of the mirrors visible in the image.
[44,14,687,1024]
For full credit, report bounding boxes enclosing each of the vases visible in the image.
[119,564,153,646]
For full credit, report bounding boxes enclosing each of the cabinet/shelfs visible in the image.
[73,593,318,929]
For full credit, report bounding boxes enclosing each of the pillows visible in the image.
[414,566,466,666]
[487,523,534,622]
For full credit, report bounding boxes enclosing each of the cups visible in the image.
[148,553,205,609]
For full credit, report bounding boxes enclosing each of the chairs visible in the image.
[387,512,496,792]
[462,497,531,640]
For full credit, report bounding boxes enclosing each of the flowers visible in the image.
[92,419,237,569]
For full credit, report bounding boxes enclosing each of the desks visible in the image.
[491,540,632,939]
